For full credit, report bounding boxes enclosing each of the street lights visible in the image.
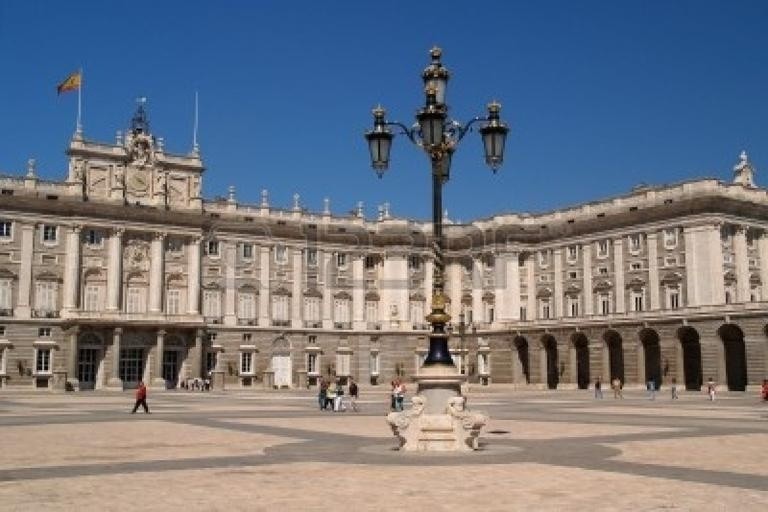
[365,40,509,368]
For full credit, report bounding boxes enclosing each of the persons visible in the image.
[320,372,329,412]
[760,378,768,401]
[130,379,149,414]
[323,375,337,411]
[611,376,623,400]
[594,375,604,399]
[390,381,398,410]
[334,383,347,412]
[162,375,211,392]
[392,376,407,411]
[670,378,679,400]
[705,376,717,401]
[347,378,361,414]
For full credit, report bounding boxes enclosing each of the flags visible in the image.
[55,68,81,95]
